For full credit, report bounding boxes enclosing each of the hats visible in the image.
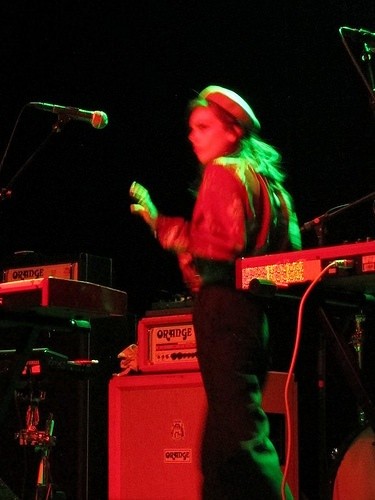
[199,85,261,134]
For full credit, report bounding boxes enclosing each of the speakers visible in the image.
[109,369,296,500]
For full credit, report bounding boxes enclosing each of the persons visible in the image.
[153,84,299,500]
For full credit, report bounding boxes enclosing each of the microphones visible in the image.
[340,24,375,48]
[28,101,108,129]
